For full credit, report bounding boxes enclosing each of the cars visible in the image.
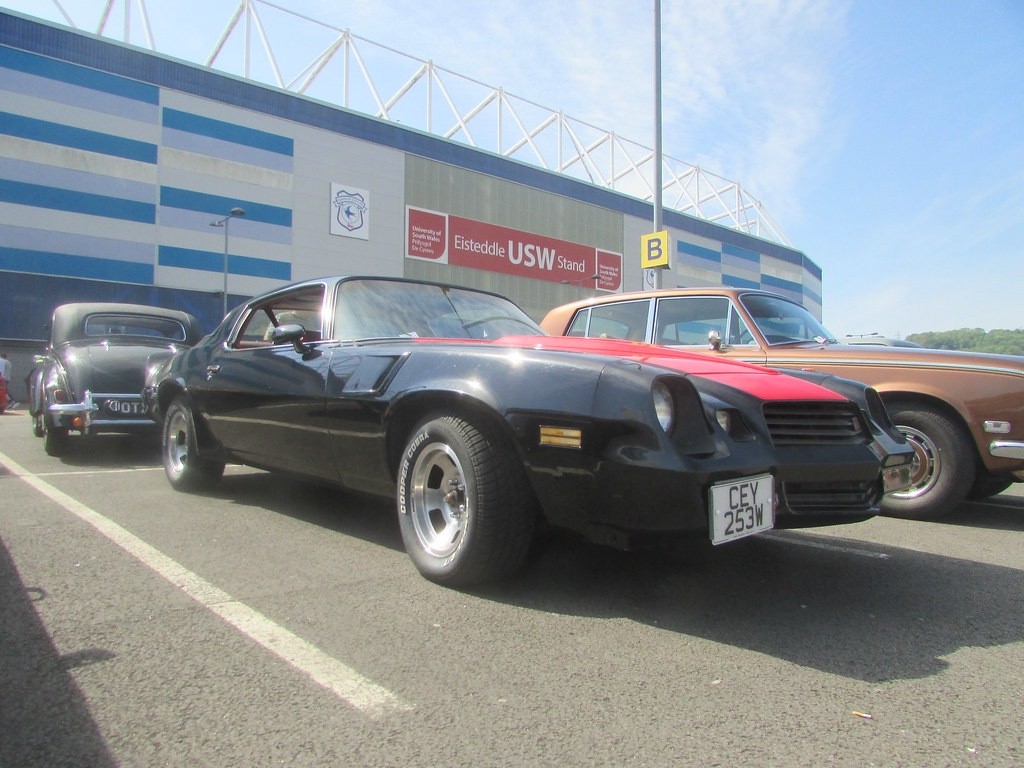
[540,287,1024,523]
[839,334,923,348]
[29,301,207,459]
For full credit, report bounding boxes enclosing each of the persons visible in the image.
[263,308,319,346]
[0,353,20,409]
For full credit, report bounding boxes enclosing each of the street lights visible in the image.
[207,205,250,320]
[560,275,601,301]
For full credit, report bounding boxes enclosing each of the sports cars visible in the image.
[149,276,920,597]
